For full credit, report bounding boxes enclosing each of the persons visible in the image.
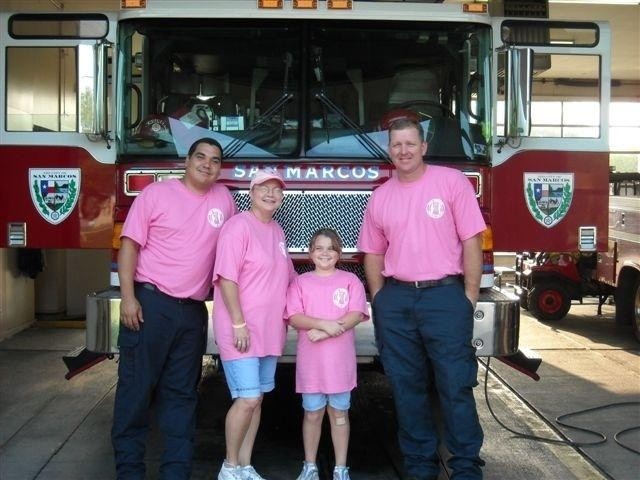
[356,118,488,479]
[211,170,298,480]
[109,137,237,479]
[283,228,370,479]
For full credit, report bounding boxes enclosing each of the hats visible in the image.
[250,171,287,191]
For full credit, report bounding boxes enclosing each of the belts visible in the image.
[143,283,159,292]
[397,276,460,288]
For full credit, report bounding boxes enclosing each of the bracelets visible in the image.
[231,318,248,328]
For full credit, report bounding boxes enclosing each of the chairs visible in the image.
[153,70,231,112]
[373,64,449,137]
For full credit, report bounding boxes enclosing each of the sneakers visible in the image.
[296,461,319,480]
[217,462,266,480]
[333,465,350,480]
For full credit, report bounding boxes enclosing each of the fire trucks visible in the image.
[503,169,640,348]
[0,1,613,384]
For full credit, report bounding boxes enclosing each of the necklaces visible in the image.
[251,208,272,224]
